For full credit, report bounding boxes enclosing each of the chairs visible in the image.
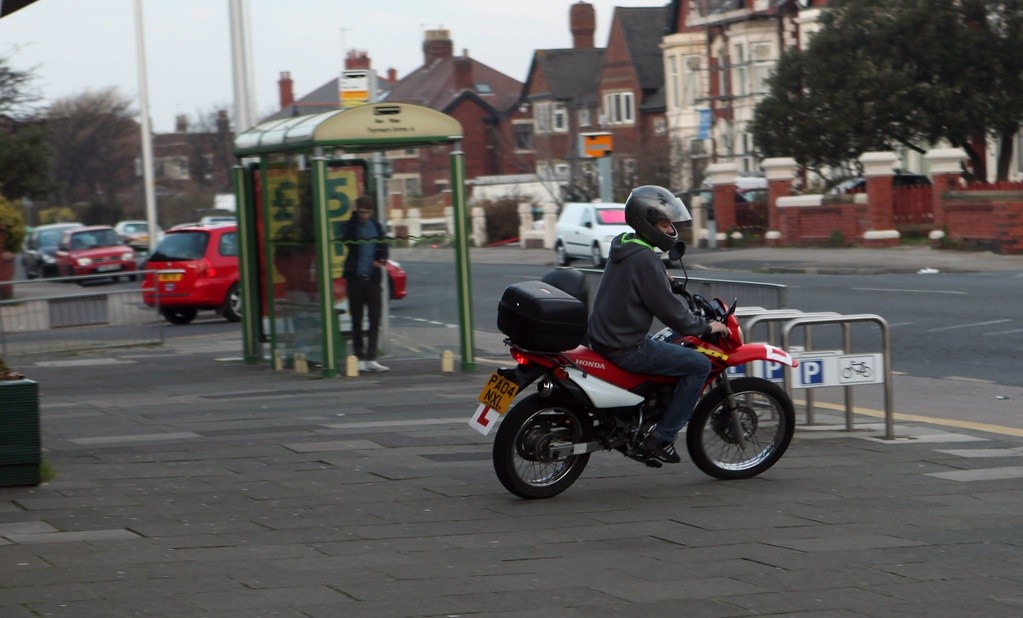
[72,238,83,246]
[96,235,106,244]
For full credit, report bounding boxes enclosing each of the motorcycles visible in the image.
[466,240,800,502]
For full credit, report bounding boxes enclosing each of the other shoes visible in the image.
[367,361,389,372]
[358,361,367,371]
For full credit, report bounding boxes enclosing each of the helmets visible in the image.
[625,185,678,252]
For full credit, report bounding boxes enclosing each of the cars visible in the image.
[20,223,85,282]
[55,226,138,287]
[673,173,935,243]
[114,218,168,259]
[140,220,409,324]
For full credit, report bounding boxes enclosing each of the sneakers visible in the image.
[644,434,681,464]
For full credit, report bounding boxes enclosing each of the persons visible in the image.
[273,207,324,369]
[341,194,390,373]
[590,185,732,464]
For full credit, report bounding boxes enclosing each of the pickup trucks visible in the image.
[542,201,679,271]
